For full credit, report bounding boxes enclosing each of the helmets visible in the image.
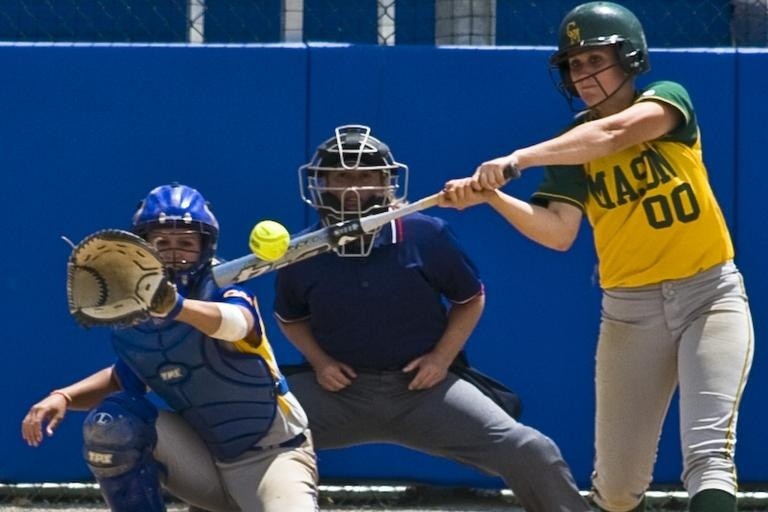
[547,0,653,113]
[131,180,222,286]
[297,124,410,259]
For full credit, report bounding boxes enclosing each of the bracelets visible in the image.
[49,388,74,405]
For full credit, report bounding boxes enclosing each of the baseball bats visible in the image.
[212,161,521,288]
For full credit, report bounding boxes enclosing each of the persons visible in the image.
[20,181,321,510]
[438,0,755,511]
[274,125,594,511]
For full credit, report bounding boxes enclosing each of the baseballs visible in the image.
[250,220,290,263]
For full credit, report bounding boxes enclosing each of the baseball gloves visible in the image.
[67,229,178,329]
